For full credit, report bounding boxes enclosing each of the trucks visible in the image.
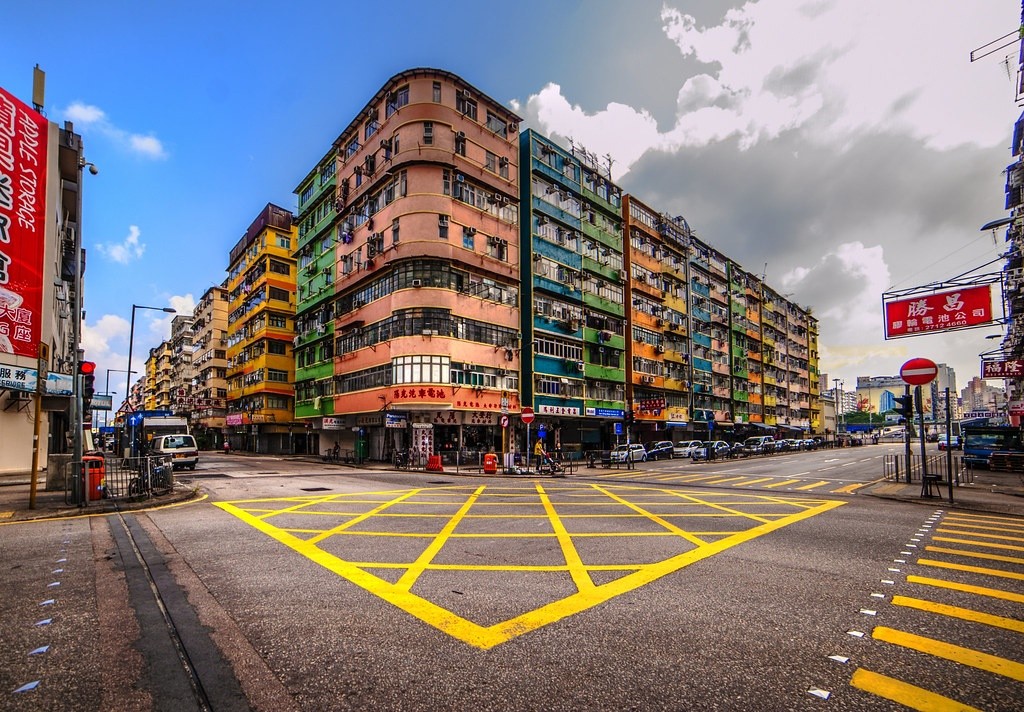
[883,425,906,437]
[105,415,190,463]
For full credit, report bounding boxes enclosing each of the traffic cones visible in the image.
[940,443,945,451]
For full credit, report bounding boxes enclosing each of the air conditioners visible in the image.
[386,91,395,101]
[466,226,476,237]
[297,139,388,291]
[501,156,509,165]
[462,363,511,375]
[299,303,363,352]
[292,375,341,390]
[534,144,741,402]
[368,108,378,117]
[492,236,508,248]
[493,193,509,206]
[457,130,465,140]
[462,89,471,101]
[412,280,422,287]
[455,174,465,183]
[510,122,516,133]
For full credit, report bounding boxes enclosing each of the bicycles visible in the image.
[128,460,168,501]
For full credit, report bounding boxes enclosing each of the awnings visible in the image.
[663,422,809,433]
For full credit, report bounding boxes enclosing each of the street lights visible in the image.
[126,304,176,410]
[96,392,117,436]
[859,393,863,439]
[105,369,138,432]
[832,378,840,434]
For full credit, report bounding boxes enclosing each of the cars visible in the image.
[674,436,820,459]
[928,432,958,450]
[644,441,674,461]
[611,444,647,464]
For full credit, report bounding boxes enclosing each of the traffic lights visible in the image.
[77,360,96,399]
[624,410,636,426]
[892,395,907,418]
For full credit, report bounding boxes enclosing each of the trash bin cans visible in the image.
[484,454,498,474]
[82,457,104,500]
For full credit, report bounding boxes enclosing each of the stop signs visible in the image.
[521,408,535,424]
[900,358,938,385]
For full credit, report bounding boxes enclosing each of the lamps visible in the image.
[378,396,385,399]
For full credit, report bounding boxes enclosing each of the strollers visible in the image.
[545,452,566,472]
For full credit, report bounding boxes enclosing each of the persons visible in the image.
[224,441,230,454]
[481,446,499,464]
[93,437,100,451]
[332,442,340,460]
[835,435,879,448]
[535,438,547,472]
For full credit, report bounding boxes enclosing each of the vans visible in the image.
[151,434,199,471]
